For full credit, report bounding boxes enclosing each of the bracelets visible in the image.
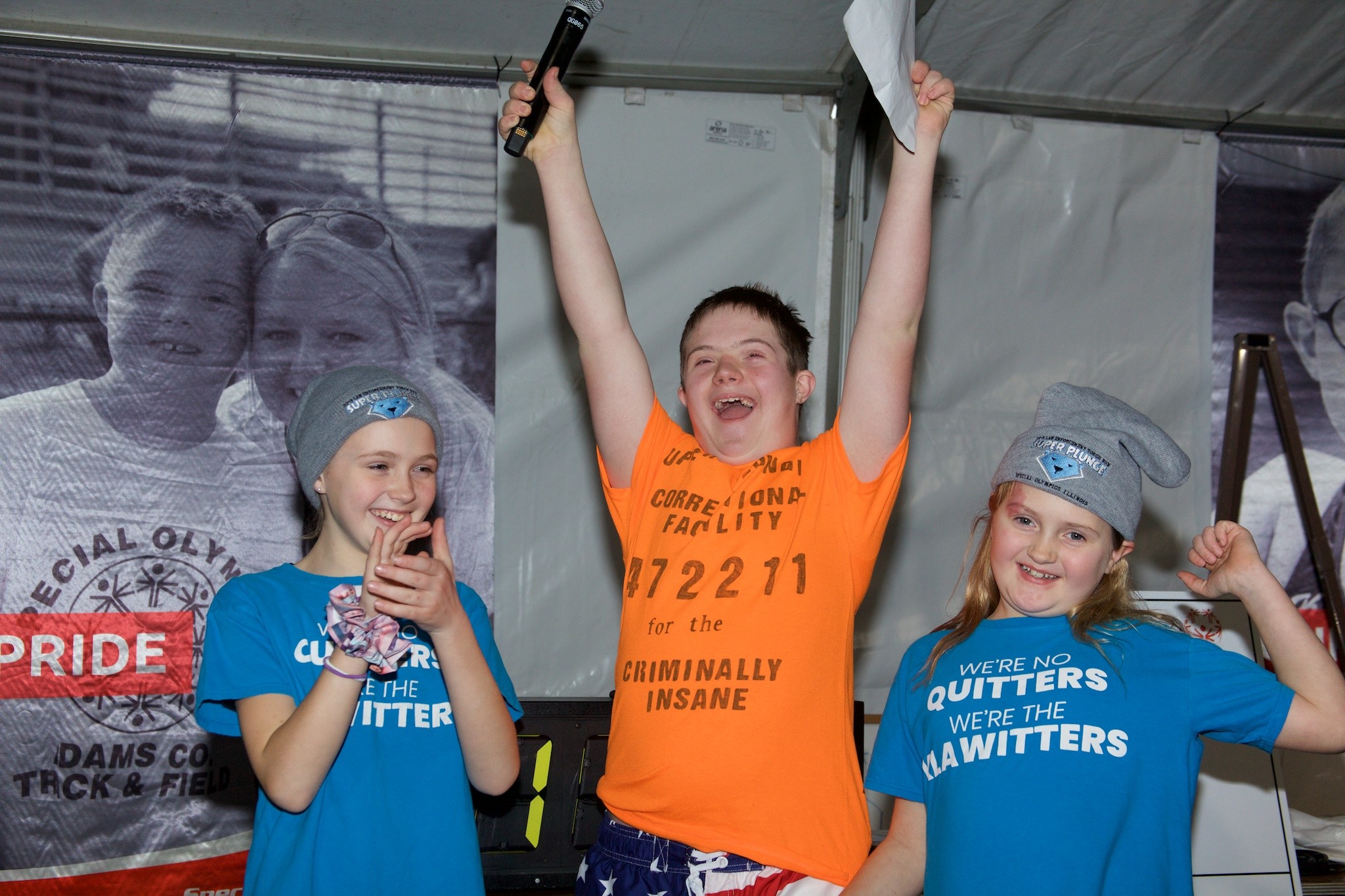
[326,583,412,674]
[323,656,367,681]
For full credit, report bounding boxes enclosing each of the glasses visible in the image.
[1310,296,1345,347]
[252,210,402,271]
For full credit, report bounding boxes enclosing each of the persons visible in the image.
[0,174,305,870]
[500,58,955,896]
[216,195,494,629]
[838,381,1345,896]
[193,366,523,896]
[1210,180,1344,875]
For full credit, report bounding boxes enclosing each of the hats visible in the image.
[991,383,1192,542]
[284,365,442,510]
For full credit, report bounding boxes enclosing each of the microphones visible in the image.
[503,0,605,157]
